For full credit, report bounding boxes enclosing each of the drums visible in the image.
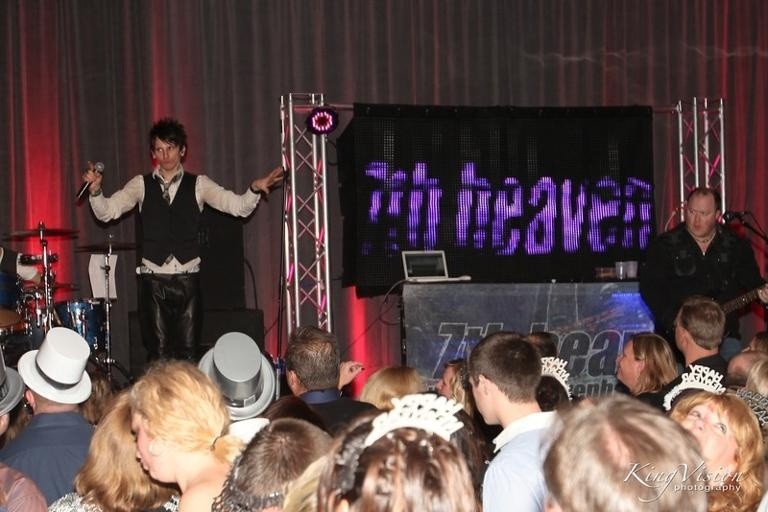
[54,296,108,356]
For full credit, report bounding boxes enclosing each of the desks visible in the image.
[400,275,658,400]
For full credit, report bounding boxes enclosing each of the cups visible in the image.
[614,261,638,280]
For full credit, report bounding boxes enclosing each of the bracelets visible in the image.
[250,185,262,194]
[91,189,101,197]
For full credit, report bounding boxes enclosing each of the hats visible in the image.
[197,331,277,423]
[0,344,26,418]
[16,324,97,408]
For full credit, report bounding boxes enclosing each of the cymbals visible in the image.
[72,242,140,253]
[7,231,80,237]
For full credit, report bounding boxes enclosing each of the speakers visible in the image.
[135,203,246,310]
[129,309,265,386]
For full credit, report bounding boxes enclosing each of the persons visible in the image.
[82,117,284,359]
[0,243,41,289]
[637,186,768,374]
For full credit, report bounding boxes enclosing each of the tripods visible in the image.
[99,253,133,392]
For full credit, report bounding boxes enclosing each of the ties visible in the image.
[154,171,182,206]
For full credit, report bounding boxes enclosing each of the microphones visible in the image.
[76,161,104,202]
[20,254,57,264]
[722,210,743,222]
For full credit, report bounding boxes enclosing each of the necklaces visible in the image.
[694,232,715,243]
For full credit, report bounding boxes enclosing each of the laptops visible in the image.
[402,250,471,283]
